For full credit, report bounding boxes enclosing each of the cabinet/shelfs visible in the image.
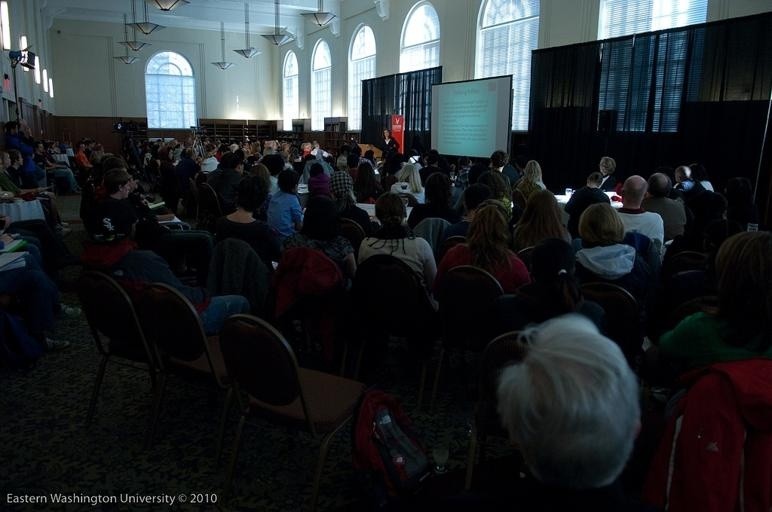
[199,118,282,148]
[278,132,360,157]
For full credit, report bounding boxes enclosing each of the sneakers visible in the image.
[45,338,69,350]
[55,302,82,320]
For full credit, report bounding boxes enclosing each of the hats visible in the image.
[328,171,353,198]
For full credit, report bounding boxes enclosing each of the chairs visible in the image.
[80,271,163,431]
[217,313,365,512]
[136,282,235,478]
[662,386,771,512]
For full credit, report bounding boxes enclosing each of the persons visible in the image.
[2,127,772,510]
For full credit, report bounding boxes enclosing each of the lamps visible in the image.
[298,1,336,27]
[112,1,189,64]
[210,20,235,72]
[260,1,294,48]
[233,1,260,59]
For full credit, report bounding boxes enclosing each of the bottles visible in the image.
[430,465,449,497]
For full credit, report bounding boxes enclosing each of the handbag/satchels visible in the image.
[0,311,44,374]
[351,383,429,512]
